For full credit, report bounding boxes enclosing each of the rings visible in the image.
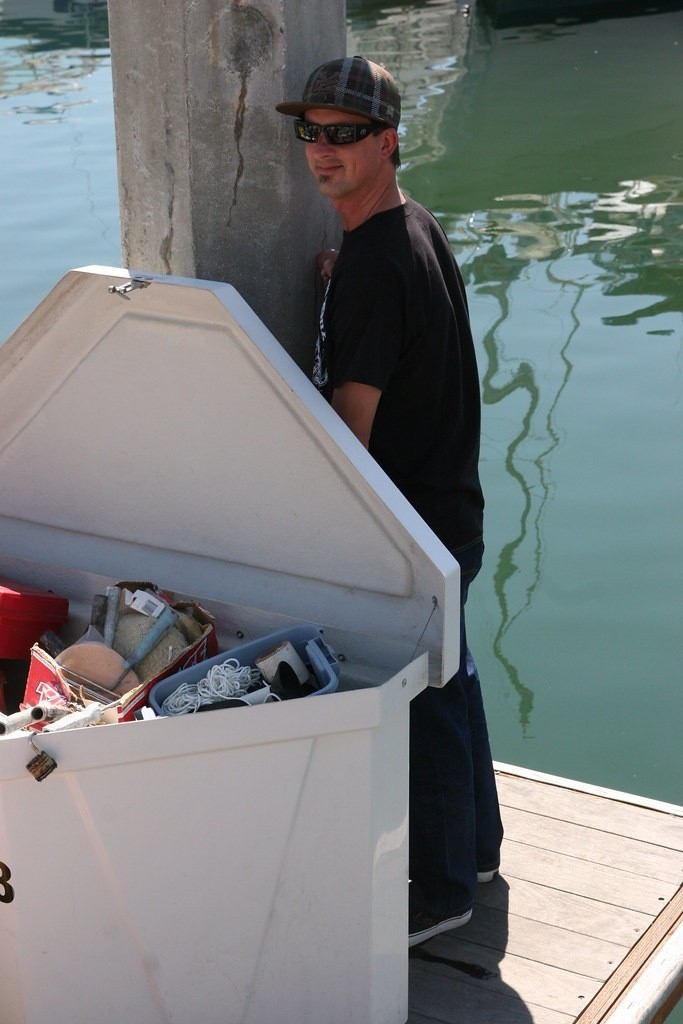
[331,249,335,251]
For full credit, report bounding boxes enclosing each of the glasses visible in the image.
[292,118,384,145]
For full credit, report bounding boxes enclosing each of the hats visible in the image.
[275,55,402,130]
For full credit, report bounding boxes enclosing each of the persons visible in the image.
[276,56,503,949]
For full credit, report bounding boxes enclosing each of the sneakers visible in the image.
[408,900,473,950]
[476,855,500,883]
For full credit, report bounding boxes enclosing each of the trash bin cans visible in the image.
[0,266,460,1024]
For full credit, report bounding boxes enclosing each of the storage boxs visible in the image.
[149,624,341,720]
[0,575,69,661]
[19,592,224,732]
